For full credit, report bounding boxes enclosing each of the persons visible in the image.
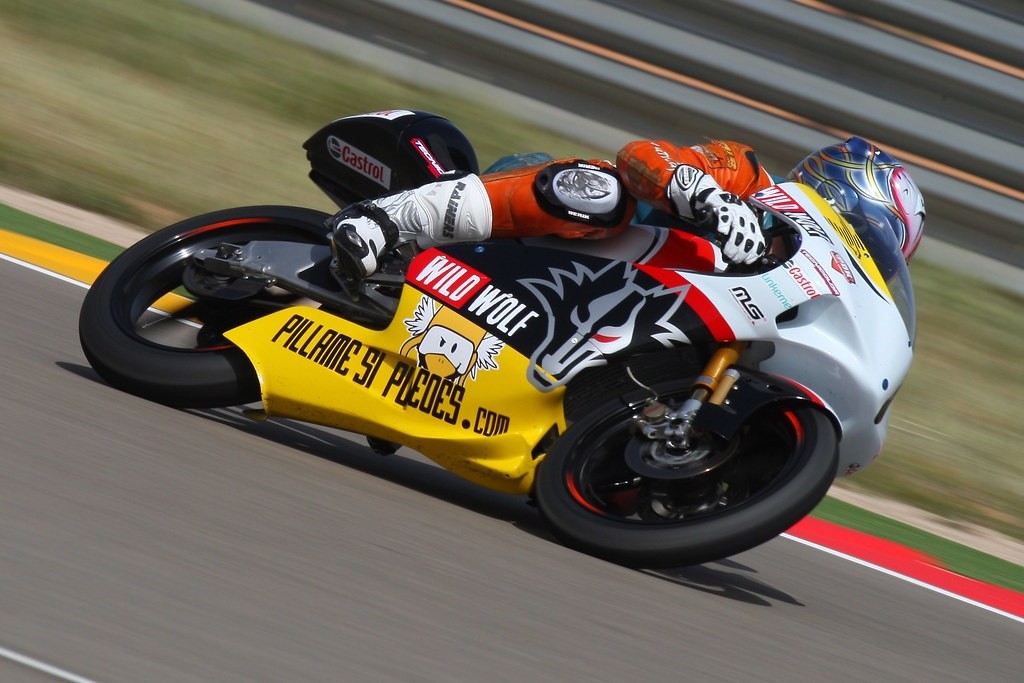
[321,136,926,456]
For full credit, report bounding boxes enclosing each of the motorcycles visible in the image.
[77,109,917,569]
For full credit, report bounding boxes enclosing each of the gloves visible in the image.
[666,163,767,265]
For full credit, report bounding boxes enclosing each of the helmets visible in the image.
[786,135,927,286]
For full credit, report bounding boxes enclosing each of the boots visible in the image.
[334,172,493,279]
